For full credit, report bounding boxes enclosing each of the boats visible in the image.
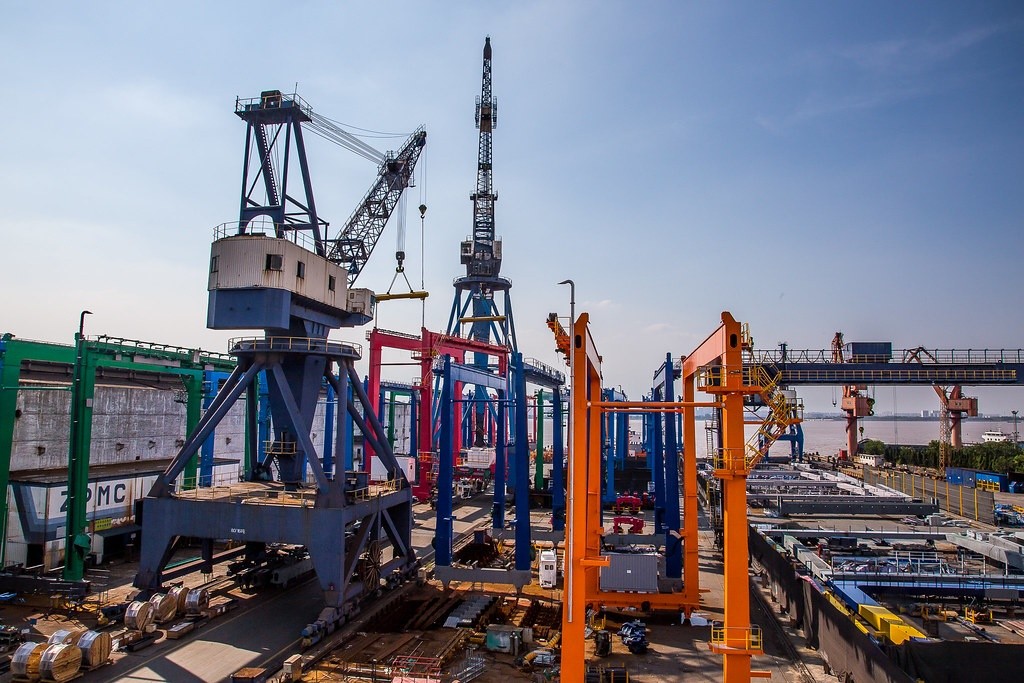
[981,427,1015,443]
